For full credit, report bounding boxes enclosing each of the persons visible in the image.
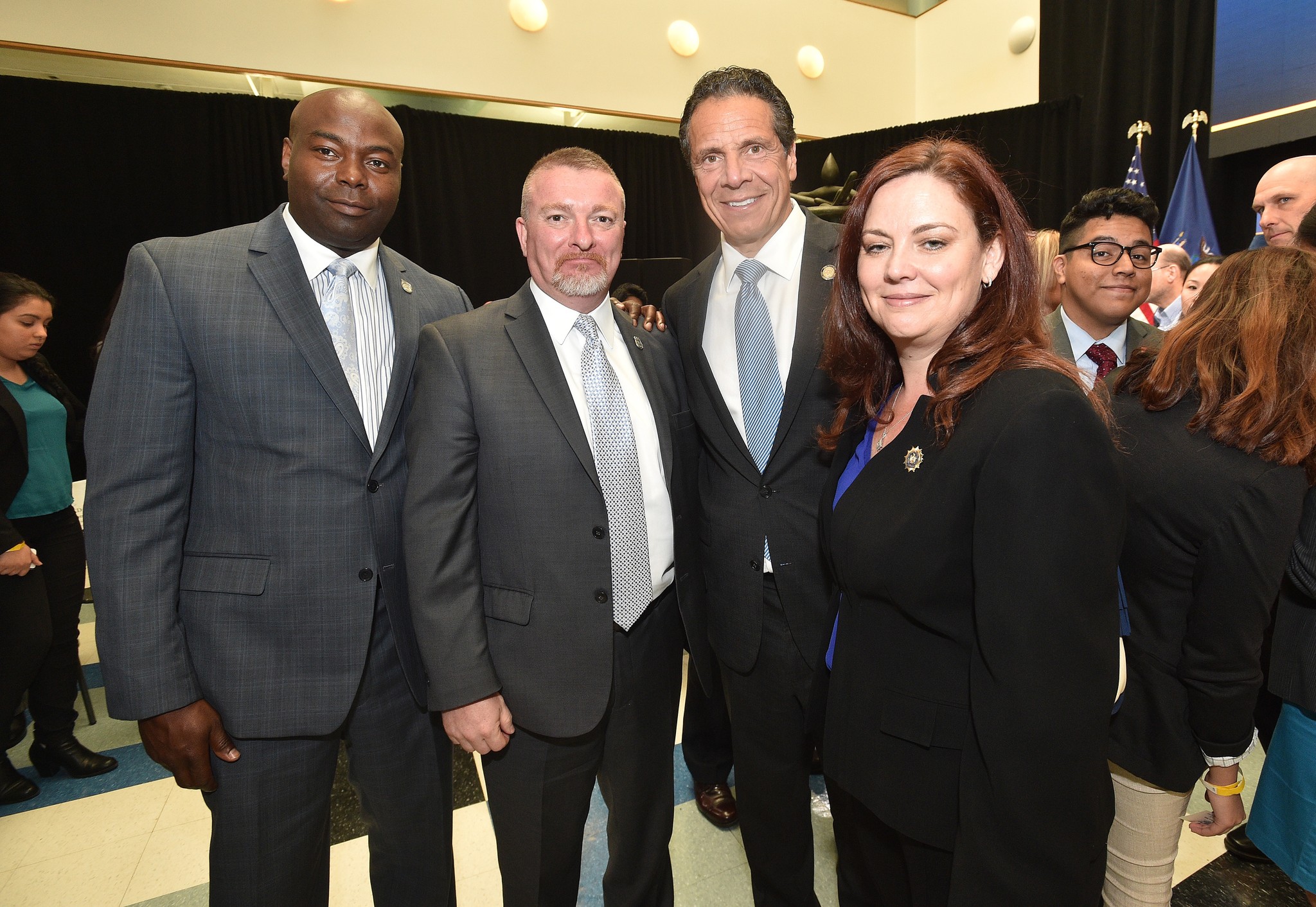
[0,271,118,806]
[664,65,903,907]
[812,136,1115,907]
[612,283,649,307]
[86,88,668,907]
[403,146,704,907]
[613,0,1316,907]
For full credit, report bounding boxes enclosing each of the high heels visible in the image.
[28,734,119,779]
[0,752,40,806]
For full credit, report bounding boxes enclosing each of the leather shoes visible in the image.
[1224,821,1276,864]
[695,783,740,830]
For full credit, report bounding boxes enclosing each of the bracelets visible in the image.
[1200,765,1245,796]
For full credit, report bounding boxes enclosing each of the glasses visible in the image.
[1062,241,1163,269]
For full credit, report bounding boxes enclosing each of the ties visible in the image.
[1086,342,1118,390]
[732,258,785,561]
[573,312,652,633]
[321,258,359,413]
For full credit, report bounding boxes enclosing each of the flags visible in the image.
[1158,109,1222,266]
[1121,118,1158,251]
